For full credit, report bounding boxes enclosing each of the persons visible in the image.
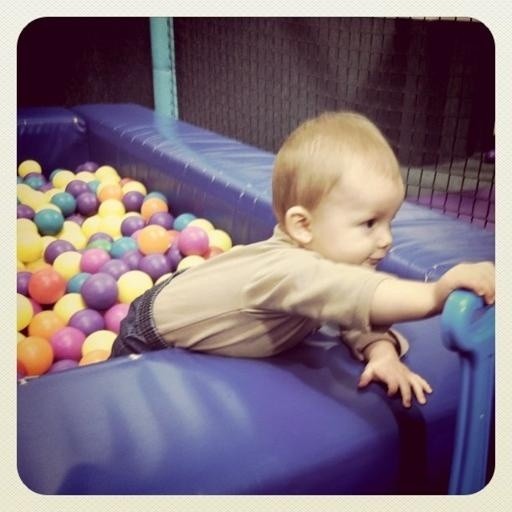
[107,109,494,409]
